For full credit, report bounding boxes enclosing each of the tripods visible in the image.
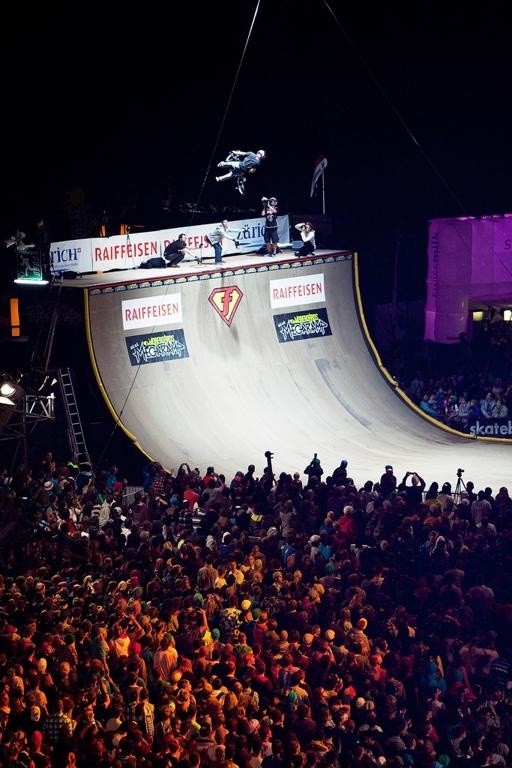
[454,478,467,502]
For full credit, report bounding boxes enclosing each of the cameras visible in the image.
[457,468,464,477]
[301,225,306,229]
[312,454,317,463]
[183,463,187,465]
[410,472,415,475]
[91,721,97,725]
[386,465,392,469]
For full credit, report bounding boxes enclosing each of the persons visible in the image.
[30,217,52,276]
[205,219,251,265]
[214,148,265,183]
[261,195,280,258]
[382,311,511,441]
[294,221,318,258]
[163,233,203,268]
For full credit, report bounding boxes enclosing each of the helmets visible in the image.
[257,150,267,159]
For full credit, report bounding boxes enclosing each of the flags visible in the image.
[309,153,328,199]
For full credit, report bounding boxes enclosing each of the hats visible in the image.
[0,451,512,768]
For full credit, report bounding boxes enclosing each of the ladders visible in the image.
[57,367,93,471]
